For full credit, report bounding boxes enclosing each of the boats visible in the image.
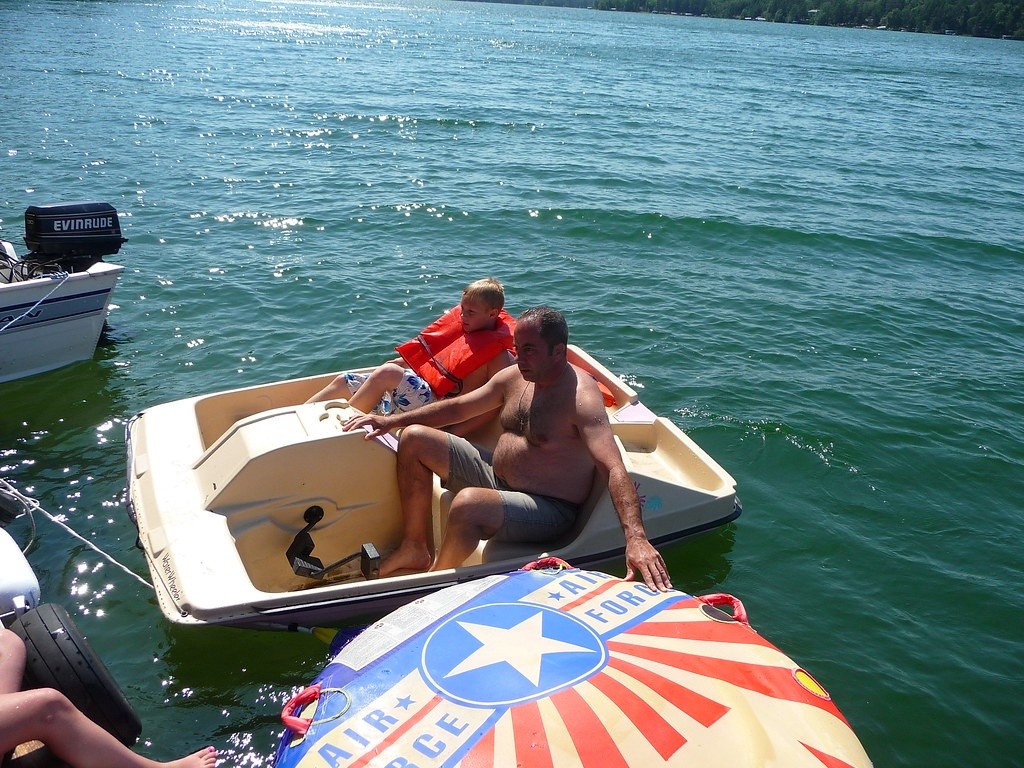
[127,346,743,627]
[0,200,131,385]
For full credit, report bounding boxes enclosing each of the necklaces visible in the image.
[518,360,568,432]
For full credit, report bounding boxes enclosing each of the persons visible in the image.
[0,625,218,768]
[301,279,511,437]
[378,303,673,595]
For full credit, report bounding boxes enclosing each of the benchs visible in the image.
[371,408,633,570]
